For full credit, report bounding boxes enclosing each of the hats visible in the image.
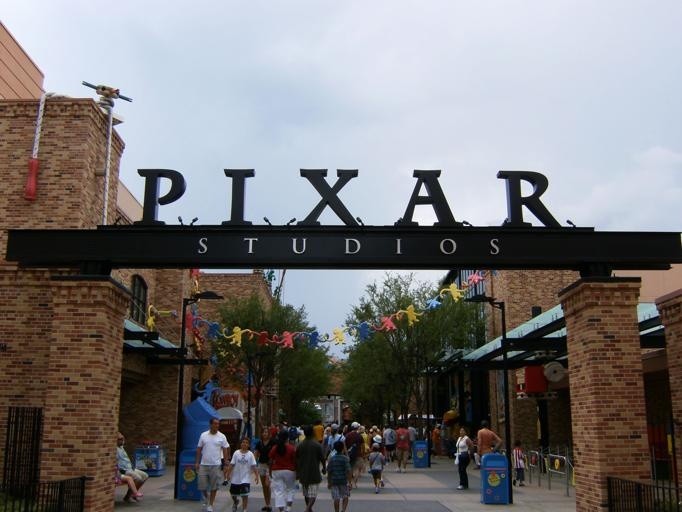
[351,422,360,429]
[331,424,337,430]
[369,425,382,449]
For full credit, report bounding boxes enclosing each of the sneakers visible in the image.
[513,480,525,486]
[124,492,144,503]
[232,501,240,512]
[375,487,379,493]
[208,504,214,512]
[381,481,385,487]
[261,506,273,512]
[457,485,464,490]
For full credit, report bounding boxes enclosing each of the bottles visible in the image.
[140,455,154,469]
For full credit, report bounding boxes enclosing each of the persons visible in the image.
[116,466,145,497]
[117,433,150,503]
[512,441,527,488]
[454,425,474,491]
[195,417,231,511]
[220,418,458,511]
[477,420,503,456]
[471,433,496,470]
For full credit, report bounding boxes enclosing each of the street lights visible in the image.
[172,287,228,499]
[246,351,268,437]
[460,292,514,501]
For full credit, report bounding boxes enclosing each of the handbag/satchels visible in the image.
[455,456,459,465]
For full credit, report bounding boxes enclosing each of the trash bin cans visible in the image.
[480,453,509,504]
[178,449,201,500]
[414,441,428,468]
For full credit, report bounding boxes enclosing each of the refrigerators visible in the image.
[134,445,167,477]
